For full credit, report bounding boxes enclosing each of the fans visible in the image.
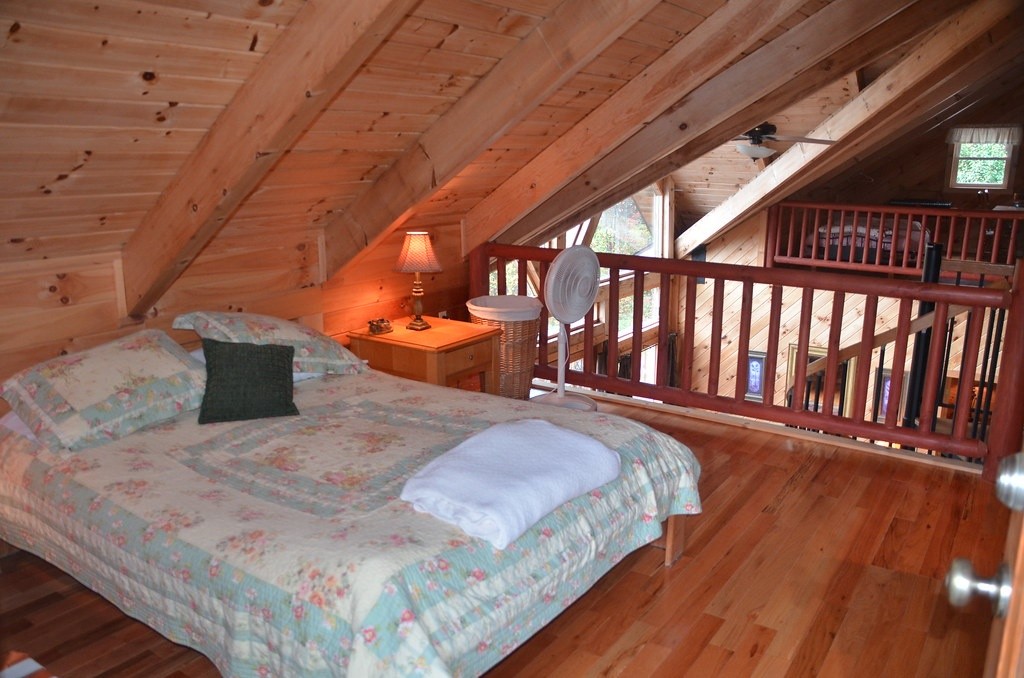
[528,245,600,412]
[731,121,838,163]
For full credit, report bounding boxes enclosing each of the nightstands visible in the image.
[345,313,504,396]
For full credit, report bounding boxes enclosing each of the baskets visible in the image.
[466,295,541,400]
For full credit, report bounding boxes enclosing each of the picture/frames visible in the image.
[871,368,910,427]
[745,351,767,402]
[785,344,856,417]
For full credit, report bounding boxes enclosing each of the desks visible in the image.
[992,205,1024,211]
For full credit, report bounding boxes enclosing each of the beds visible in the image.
[806,218,931,261]
[0,346,688,678]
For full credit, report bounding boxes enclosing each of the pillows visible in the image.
[0,328,207,453]
[197,338,300,424]
[172,311,373,375]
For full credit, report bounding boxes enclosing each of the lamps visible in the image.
[391,232,443,331]
